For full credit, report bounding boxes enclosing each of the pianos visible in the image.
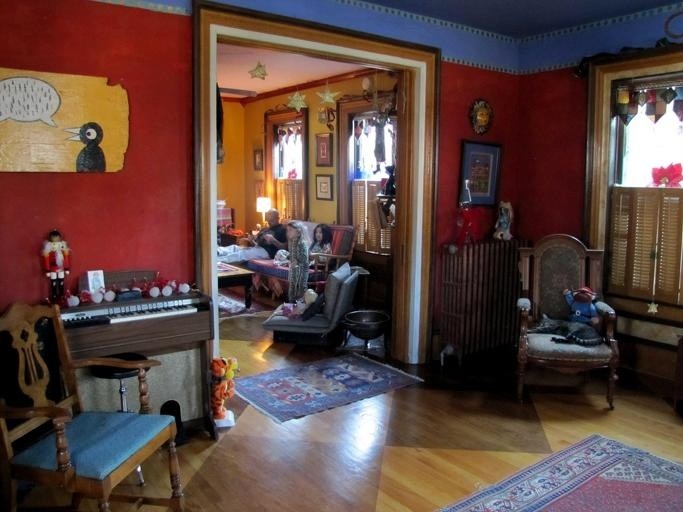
[59,290,217,442]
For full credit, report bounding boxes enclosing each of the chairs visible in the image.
[515,234,619,410]
[0,300,183,512]
[262,270,359,344]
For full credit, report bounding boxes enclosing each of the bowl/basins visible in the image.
[217,203,225,208]
[251,230,259,236]
[344,310,390,341]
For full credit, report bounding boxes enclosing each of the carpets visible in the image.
[234,352,424,425]
[437,433,683,512]
[218,288,275,323]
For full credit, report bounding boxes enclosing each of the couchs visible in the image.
[239,224,358,300]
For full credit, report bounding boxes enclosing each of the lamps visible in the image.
[256,197,270,227]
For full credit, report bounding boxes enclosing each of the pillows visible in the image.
[324,262,351,320]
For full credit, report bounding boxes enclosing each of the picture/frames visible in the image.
[315,174,333,200]
[315,133,333,167]
[458,139,503,208]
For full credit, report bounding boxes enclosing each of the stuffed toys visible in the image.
[272,288,318,318]
[563,286,604,345]
[493,201,514,240]
[211,357,240,427]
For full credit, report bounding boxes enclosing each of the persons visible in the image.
[217,208,288,264]
[42,229,71,301]
[273,223,333,268]
[287,221,309,301]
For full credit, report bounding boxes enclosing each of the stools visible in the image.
[85,352,150,487]
